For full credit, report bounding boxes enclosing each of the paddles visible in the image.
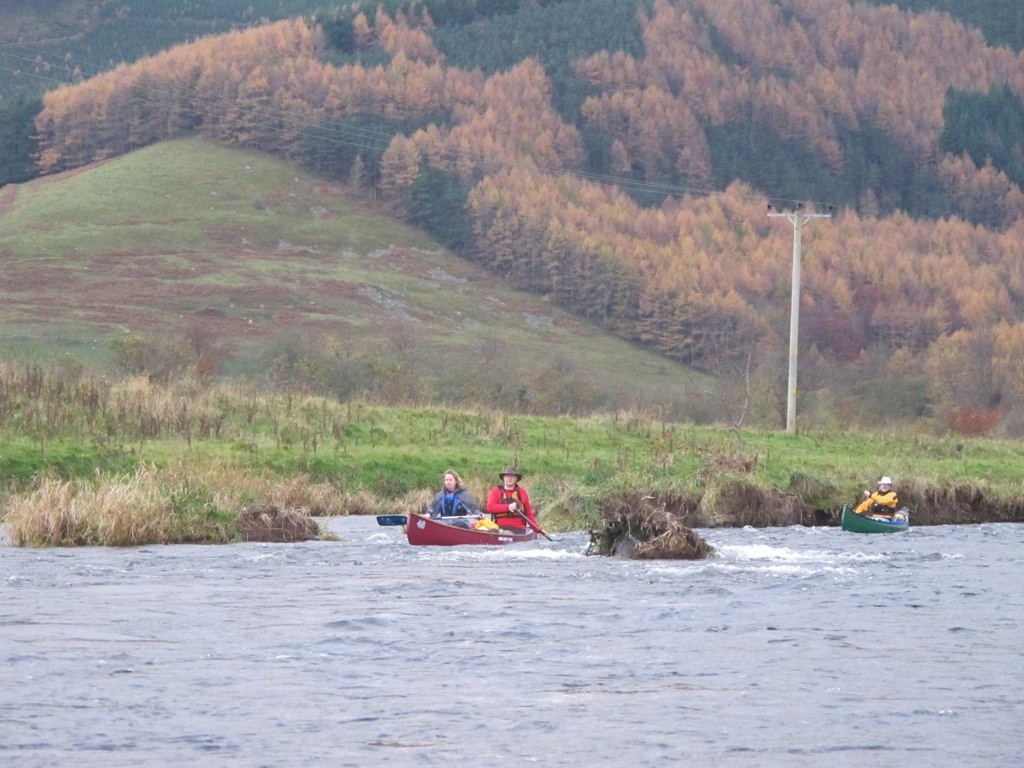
[377,514,482,526]
[515,508,552,541]
[854,494,866,510]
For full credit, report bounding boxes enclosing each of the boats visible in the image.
[840,503,910,534]
[406,510,538,546]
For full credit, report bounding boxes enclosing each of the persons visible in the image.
[486,467,549,538]
[422,469,485,530]
[853,476,899,525]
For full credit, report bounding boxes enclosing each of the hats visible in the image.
[498,468,522,482]
[878,477,893,486]
